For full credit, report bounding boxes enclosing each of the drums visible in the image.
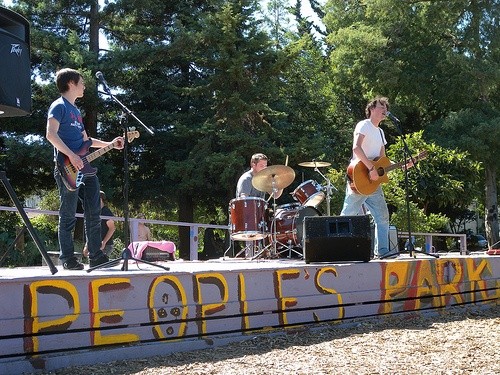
[290,178,326,208]
[228,196,268,241]
[266,203,323,257]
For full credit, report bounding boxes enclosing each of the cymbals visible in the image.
[298,162,332,167]
[251,164,295,191]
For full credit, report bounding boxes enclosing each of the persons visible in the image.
[83,191,115,259]
[130,212,151,243]
[46,67,125,271]
[235,153,282,260]
[203,220,224,259]
[340,97,416,259]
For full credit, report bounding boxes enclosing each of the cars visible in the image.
[456,235,487,250]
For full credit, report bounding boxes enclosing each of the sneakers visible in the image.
[63,258,84,270]
[90,256,120,268]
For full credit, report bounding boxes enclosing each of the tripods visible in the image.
[380,122,439,259]
[86,82,171,273]
[249,175,303,261]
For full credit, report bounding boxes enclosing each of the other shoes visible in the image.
[374,252,397,259]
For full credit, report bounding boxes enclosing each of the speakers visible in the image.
[0,5,32,117]
[303,214,375,264]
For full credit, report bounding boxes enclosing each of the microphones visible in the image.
[96,71,105,83]
[386,111,400,122]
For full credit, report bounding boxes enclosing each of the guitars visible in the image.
[346,149,428,196]
[56,126,140,191]
